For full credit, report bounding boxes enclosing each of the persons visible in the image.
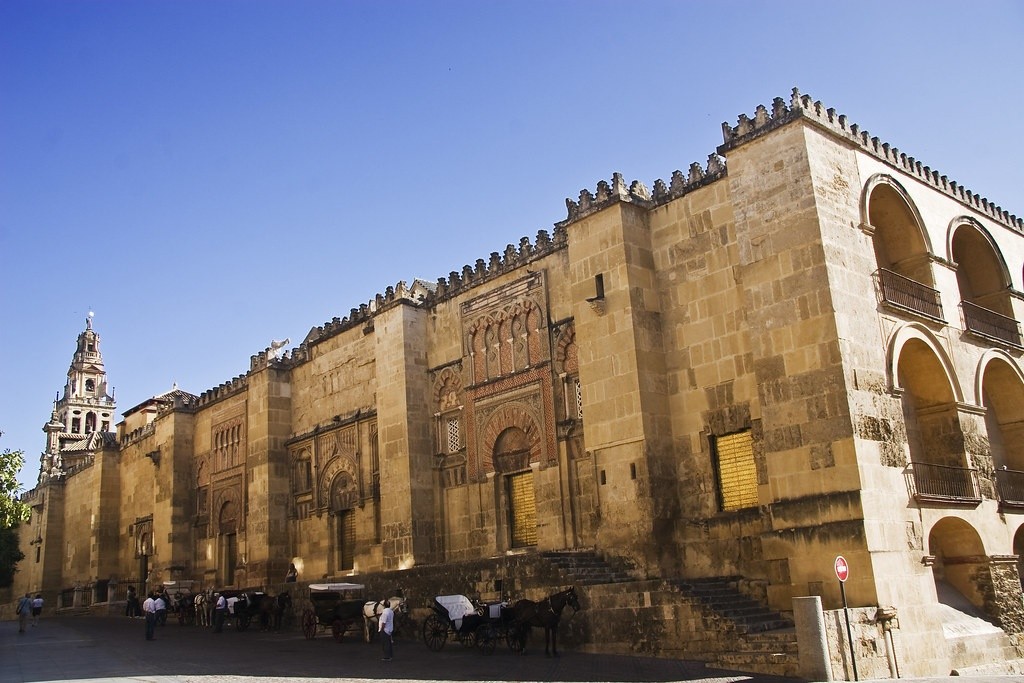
[378,601,394,662]
[212,592,226,634]
[285,563,297,583]
[16,592,32,633]
[143,590,167,641]
[125,585,140,619]
[31,594,44,627]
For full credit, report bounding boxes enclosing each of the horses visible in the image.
[363,596,408,643]
[258,590,292,634]
[194,585,217,627]
[513,585,581,658]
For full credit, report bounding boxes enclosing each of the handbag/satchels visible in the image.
[286,572,293,578]
[16,611,21,615]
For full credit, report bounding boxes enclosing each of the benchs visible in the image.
[434,594,475,623]
[310,592,362,621]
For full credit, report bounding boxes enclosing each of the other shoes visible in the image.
[128,616,133,618]
[151,637,157,640]
[381,658,392,661]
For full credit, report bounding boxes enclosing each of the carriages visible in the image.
[158,586,216,629]
[423,586,581,658]
[222,589,293,633]
[301,583,407,644]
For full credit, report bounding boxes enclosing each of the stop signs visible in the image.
[834,556,849,582]
